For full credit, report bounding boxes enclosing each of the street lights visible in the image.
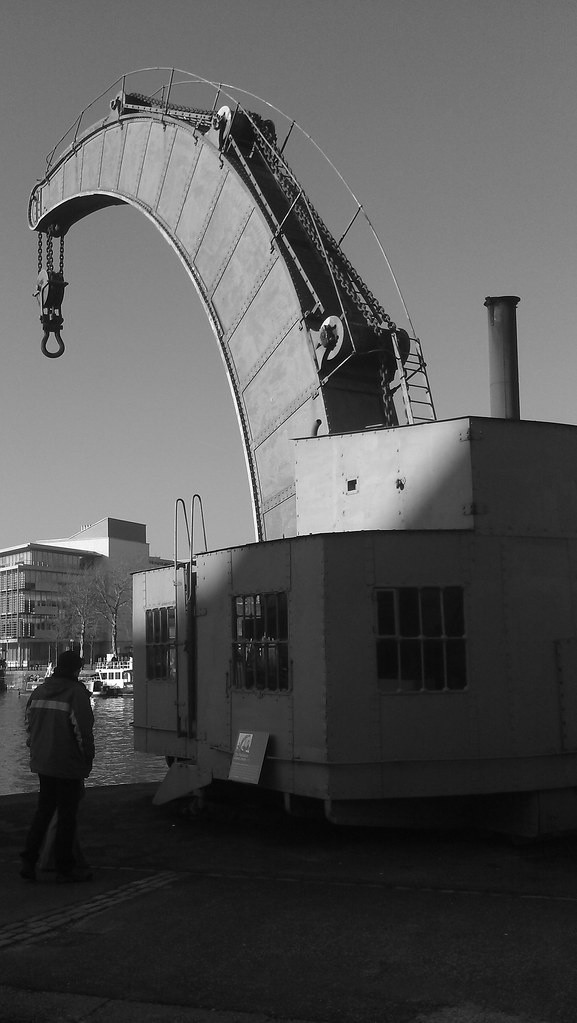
[56,639,74,665]
[6,640,9,666]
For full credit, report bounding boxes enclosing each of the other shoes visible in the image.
[19,861,37,880]
[55,871,80,882]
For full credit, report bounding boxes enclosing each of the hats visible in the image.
[58,651,81,672]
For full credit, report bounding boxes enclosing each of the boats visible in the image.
[20,657,134,698]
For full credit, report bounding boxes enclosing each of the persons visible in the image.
[19,650,95,884]
